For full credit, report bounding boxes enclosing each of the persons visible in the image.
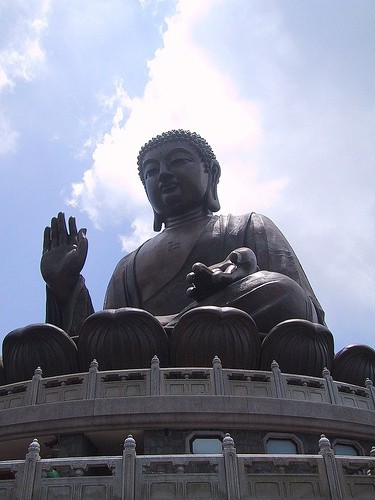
[40,130,329,345]
[367,448,375,475]
